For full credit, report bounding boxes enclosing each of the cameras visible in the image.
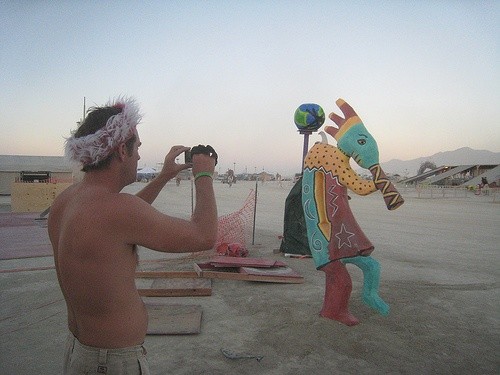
[185,151,193,165]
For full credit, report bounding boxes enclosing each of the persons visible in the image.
[481,176,489,187]
[228,173,283,187]
[47,95,218,375]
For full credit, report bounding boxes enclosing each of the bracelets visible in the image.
[194,171,215,182]
